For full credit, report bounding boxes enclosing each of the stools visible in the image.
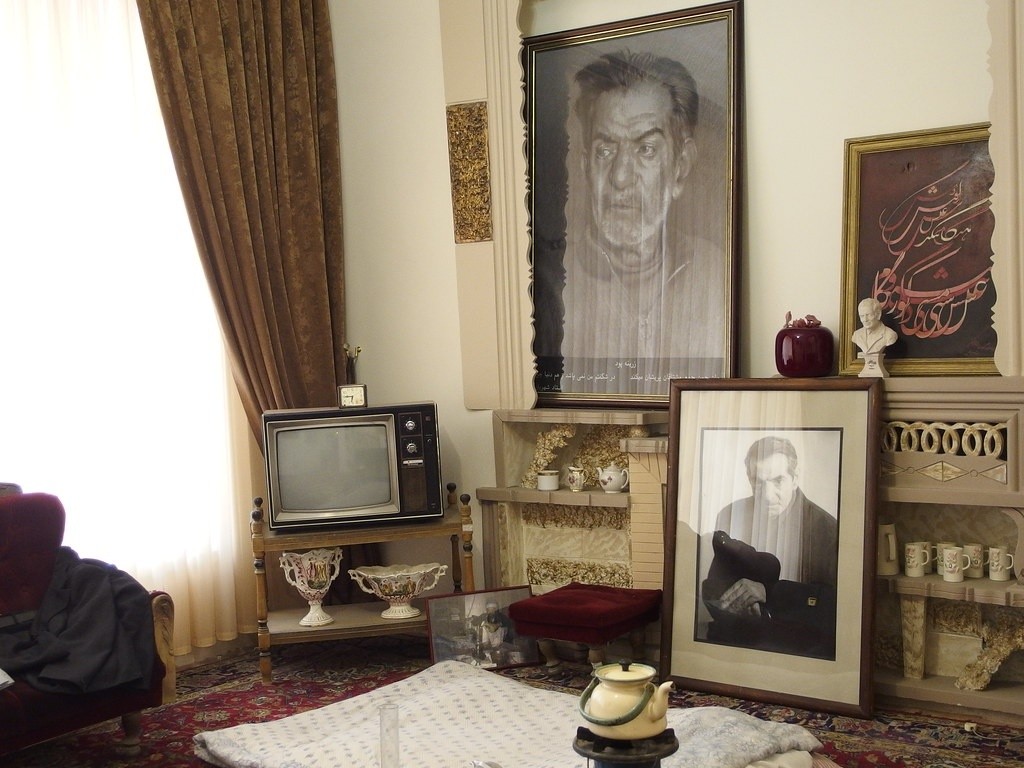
[508,581,664,685]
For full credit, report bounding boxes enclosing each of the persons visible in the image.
[471,600,509,648]
[852,298,898,354]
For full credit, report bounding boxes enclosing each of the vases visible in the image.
[280,547,344,628]
[348,562,449,621]
[775,326,835,378]
[658,378,884,720]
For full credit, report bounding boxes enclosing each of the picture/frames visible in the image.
[521,0,745,411]
[836,121,1006,379]
[422,585,545,671]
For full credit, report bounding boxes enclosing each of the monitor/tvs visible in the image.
[261,400,444,531]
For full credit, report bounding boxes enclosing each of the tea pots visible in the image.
[596,461,628,494]
[578,659,675,741]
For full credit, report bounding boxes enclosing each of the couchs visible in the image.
[0,481,176,758]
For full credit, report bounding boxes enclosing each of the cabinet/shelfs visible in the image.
[250,482,475,687]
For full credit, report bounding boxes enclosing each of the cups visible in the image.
[905,541,937,578]
[537,470,558,491]
[963,542,989,577]
[988,547,1013,581]
[279,547,344,626]
[937,541,970,582]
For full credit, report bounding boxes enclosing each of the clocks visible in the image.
[337,385,367,408]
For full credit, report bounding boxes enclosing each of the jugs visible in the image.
[567,467,587,492]
[877,523,899,576]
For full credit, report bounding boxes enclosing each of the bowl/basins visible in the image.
[347,562,448,620]
